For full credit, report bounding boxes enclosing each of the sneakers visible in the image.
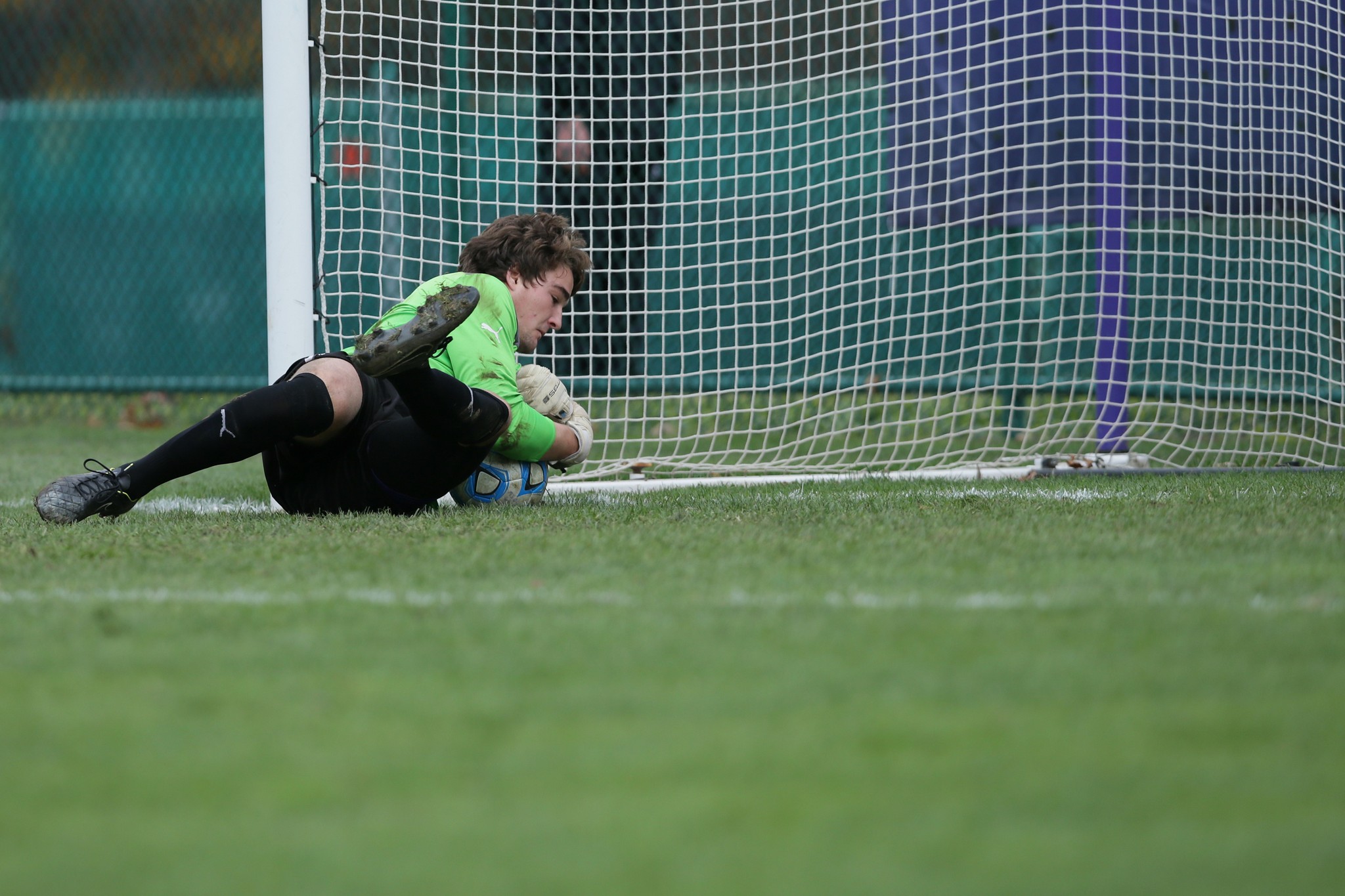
[353,284,479,377]
[33,458,139,527]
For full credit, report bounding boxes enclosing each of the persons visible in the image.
[528,0,681,375]
[32,213,594,525]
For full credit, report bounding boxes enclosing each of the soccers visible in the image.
[449,451,549,508]
